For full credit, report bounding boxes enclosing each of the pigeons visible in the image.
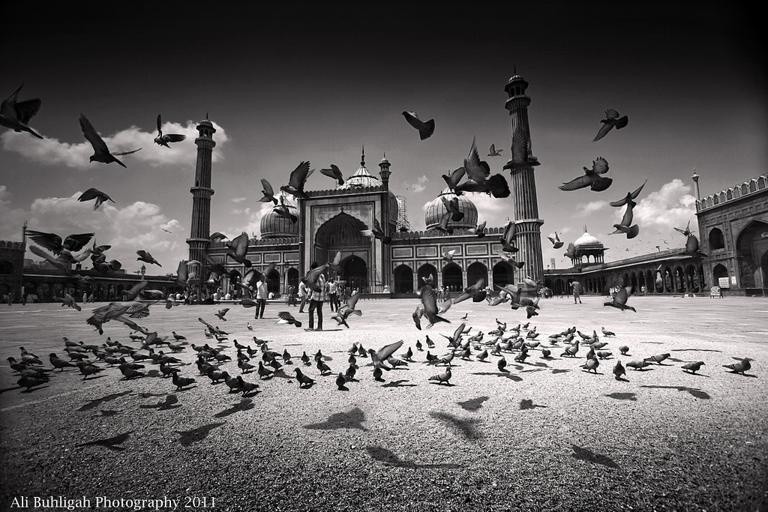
[722,356,752,375]
[0,81,709,398]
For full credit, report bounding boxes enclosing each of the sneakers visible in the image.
[260,317,265,319]
[573,301,582,304]
[255,317,258,319]
[315,328,322,331]
[303,327,314,331]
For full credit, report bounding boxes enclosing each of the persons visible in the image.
[439,284,462,299]
[569,279,582,304]
